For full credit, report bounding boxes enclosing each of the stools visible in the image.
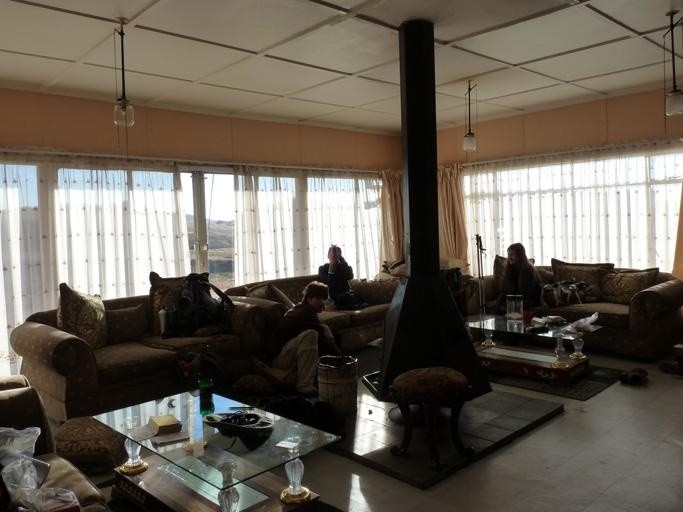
[234,374,281,396]
[55,415,127,466]
[389,367,476,471]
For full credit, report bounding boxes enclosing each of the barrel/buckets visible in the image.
[319,355,358,404]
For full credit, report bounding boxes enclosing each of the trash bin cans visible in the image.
[316,355,358,416]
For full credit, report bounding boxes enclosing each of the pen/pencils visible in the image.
[229,407,254,408]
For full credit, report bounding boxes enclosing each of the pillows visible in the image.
[101,303,149,342]
[491,254,535,278]
[263,282,297,309]
[492,260,552,296]
[243,280,269,299]
[147,271,213,336]
[55,280,109,350]
[248,355,295,388]
[596,266,660,306]
[550,258,615,298]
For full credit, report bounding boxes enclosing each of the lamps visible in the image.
[112,17,134,127]
[662,10,682,117]
[462,79,477,152]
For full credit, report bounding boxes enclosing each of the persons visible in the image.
[317,244,361,313]
[252,279,347,400]
[491,243,545,315]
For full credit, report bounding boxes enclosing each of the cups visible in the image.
[197,378,214,415]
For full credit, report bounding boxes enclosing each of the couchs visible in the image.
[8,273,268,446]
[475,261,683,364]
[0,387,112,510]
[219,274,397,351]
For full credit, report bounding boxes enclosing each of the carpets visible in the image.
[491,361,629,402]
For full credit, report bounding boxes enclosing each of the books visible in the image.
[125,413,182,443]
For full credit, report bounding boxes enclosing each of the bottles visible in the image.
[507,320,523,334]
[506,293,523,318]
